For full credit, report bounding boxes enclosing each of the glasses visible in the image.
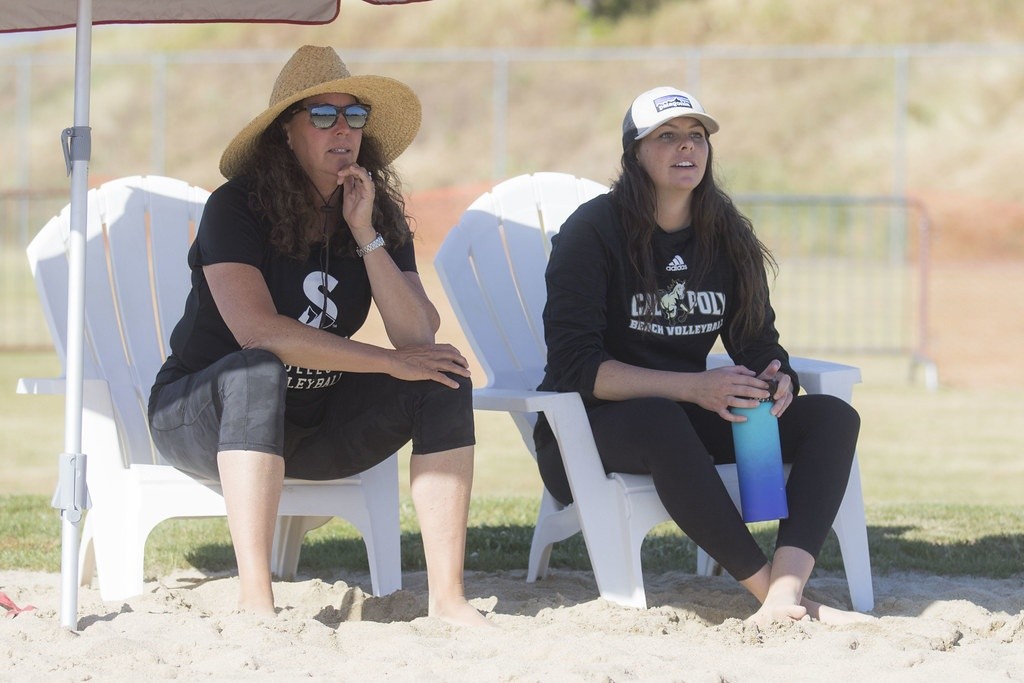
[289,103,371,130]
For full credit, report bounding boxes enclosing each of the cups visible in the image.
[725,378,789,524]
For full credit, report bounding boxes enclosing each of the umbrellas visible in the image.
[0,1,432,628]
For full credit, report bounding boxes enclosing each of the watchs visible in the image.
[356,232,387,257]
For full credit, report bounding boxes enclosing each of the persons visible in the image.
[147,44,499,630]
[534,87,877,623]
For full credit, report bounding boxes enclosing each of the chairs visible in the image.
[16,174,402,604]
[435,171,874,613]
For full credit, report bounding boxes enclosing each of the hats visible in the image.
[621,86,720,156]
[219,45,423,181]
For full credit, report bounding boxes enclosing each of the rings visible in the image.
[367,172,372,180]
